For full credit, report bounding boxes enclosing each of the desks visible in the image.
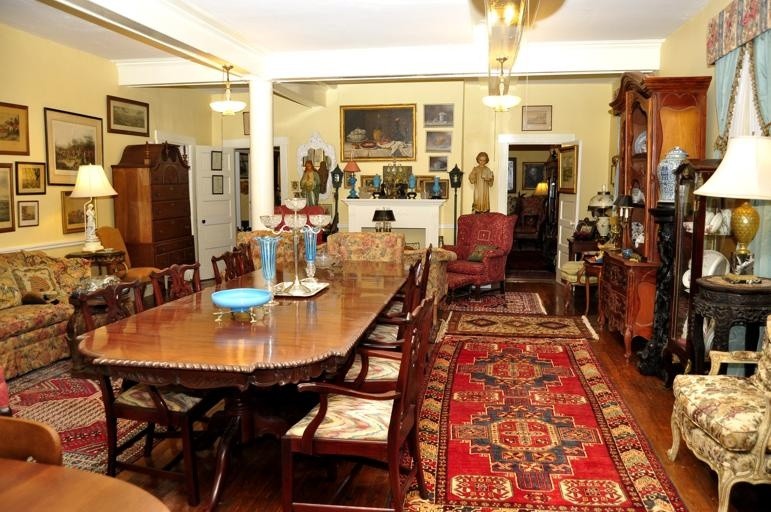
[565,236,602,259]
[688,276,770,374]
[72,261,409,478]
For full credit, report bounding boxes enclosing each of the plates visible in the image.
[211,287,271,312]
[271,281,330,298]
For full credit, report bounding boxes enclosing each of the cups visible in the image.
[622,249,632,259]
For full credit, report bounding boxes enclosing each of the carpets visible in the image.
[451,292,545,314]
[442,308,599,340]
[401,336,685,512]
[3,371,204,476]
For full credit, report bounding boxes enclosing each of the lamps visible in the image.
[345,163,360,199]
[586,185,614,237]
[533,177,550,197]
[475,0,536,112]
[209,62,246,119]
[329,164,342,223]
[690,134,769,283]
[69,163,117,251]
[448,164,462,245]
[372,208,394,232]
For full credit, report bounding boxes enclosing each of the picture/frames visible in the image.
[16,161,45,195]
[522,104,552,130]
[422,104,454,128]
[361,175,380,191]
[557,145,577,195]
[0,164,16,232]
[60,190,95,233]
[338,102,416,162]
[107,94,150,137]
[43,107,105,186]
[0,102,30,156]
[415,176,434,192]
[16,200,40,227]
[424,130,452,152]
[430,155,448,171]
[522,161,546,190]
[210,150,223,171]
[508,156,516,193]
[422,179,448,198]
[212,175,224,195]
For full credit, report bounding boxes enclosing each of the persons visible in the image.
[469,153,493,215]
[86,203,95,236]
[300,160,320,207]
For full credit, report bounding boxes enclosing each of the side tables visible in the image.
[65,249,128,283]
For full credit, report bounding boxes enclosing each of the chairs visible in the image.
[211,251,244,282]
[2,420,60,464]
[561,245,610,314]
[667,314,771,507]
[281,294,437,512]
[75,288,220,506]
[327,297,435,395]
[93,226,160,303]
[382,243,433,321]
[145,259,202,305]
[233,239,256,275]
[362,261,422,354]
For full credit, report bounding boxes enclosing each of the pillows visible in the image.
[15,265,60,301]
[0,268,23,310]
[468,244,497,262]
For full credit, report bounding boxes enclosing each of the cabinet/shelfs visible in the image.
[674,157,741,380]
[600,72,712,359]
[112,143,196,267]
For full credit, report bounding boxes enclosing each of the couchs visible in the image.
[318,231,447,319]
[440,212,516,298]
[237,230,453,324]
[513,195,545,248]
[0,250,135,383]
